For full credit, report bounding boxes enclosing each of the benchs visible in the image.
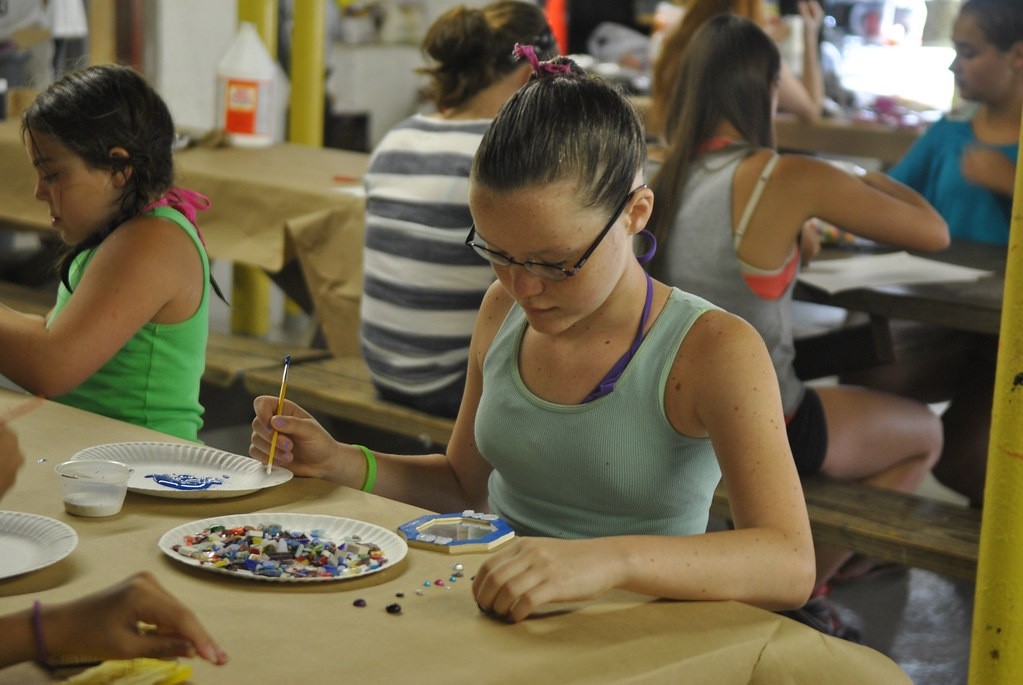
[0,296,979,578]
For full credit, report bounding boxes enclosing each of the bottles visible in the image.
[215,19,287,151]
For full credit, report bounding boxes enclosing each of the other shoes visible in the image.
[795,584,862,642]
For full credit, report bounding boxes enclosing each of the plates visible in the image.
[70,442,294,499]
[158,512,408,583]
[0,510,79,579]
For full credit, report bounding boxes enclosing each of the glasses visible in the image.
[465,183,647,281]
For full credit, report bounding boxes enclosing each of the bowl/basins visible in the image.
[55,460,135,517]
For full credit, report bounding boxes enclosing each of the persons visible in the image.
[250,42,818,623]
[0,0,231,444]
[358,0,1022,643]
[0,421,25,501]
[0,570,230,670]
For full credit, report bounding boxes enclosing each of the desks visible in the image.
[0,385,913,684]
[794,232,1006,400]
[630,93,929,165]
[0,110,372,361]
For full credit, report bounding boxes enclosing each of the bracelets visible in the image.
[354,444,378,493]
[33,601,47,661]
